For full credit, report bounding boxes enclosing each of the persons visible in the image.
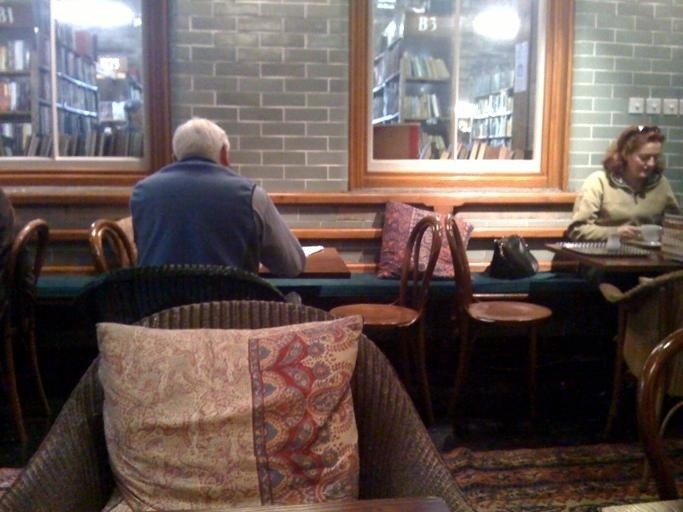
[564,124,683,284]
[128,117,305,277]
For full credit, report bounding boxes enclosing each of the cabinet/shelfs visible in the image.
[371,0,513,160]
[0,0,144,157]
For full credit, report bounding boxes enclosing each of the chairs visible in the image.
[2,300,472,509]
[1,219,136,446]
[596,267,683,498]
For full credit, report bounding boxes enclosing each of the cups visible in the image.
[637,224,662,240]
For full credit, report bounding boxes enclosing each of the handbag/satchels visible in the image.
[482,237,538,279]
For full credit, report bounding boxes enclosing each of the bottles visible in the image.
[605,234,621,250]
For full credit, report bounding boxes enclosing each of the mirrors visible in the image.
[0,0,169,186]
[347,0,573,195]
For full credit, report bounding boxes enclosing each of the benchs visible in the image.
[0,189,578,299]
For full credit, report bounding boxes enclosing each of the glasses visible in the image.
[621,125,661,135]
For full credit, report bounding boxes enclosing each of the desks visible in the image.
[259,245,349,279]
[541,238,683,273]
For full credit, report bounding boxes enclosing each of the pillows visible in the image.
[92,311,366,506]
[380,198,471,280]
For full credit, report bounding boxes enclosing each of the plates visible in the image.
[634,241,660,249]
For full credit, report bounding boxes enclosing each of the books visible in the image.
[371,0,516,159]
[659,211,683,265]
[0,2,143,155]
